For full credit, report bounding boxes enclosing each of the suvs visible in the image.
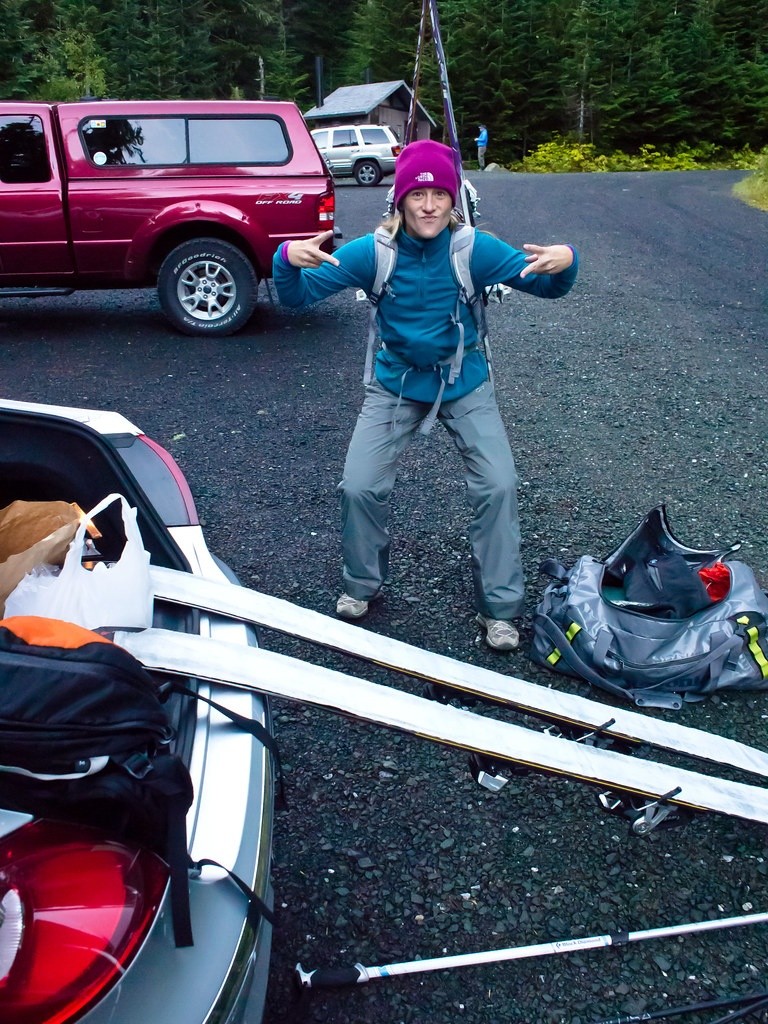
[308,123,406,187]
[0,100,343,333]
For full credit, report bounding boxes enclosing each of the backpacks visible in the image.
[0,614,194,845]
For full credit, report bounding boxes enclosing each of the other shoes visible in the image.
[477,166,484,171]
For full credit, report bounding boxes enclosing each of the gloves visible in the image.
[474,138,478,141]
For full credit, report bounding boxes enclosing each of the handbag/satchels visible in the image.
[0,500,79,619]
[5,494,154,628]
[530,504,768,710]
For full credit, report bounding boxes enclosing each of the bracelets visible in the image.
[282,242,289,260]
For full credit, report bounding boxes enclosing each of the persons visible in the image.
[474,124,488,172]
[272,140,579,650]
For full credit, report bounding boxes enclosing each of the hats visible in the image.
[478,124,486,128]
[395,140,457,211]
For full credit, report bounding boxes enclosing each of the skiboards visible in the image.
[112,564,768,837]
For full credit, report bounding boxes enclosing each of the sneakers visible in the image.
[476,612,519,651]
[336,591,369,618]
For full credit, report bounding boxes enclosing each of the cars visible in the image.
[0,399,279,1024]
